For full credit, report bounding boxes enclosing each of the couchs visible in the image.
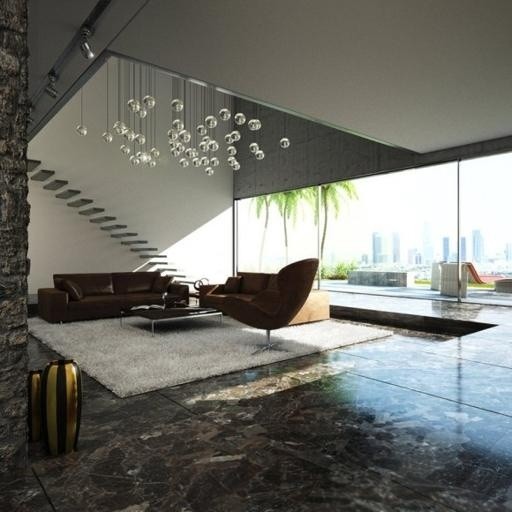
[200,272,330,325]
[38,272,189,325]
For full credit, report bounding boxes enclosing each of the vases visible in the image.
[28,371,44,442]
[44,359,82,454]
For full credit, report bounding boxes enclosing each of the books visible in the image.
[131,304,166,311]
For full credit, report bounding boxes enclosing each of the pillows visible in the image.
[152,276,172,292]
[54,278,80,301]
[225,276,242,293]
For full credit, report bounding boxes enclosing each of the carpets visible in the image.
[27,308,393,398]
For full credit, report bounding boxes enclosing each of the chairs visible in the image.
[217,258,320,355]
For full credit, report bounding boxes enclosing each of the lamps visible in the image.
[27,0,118,133]
